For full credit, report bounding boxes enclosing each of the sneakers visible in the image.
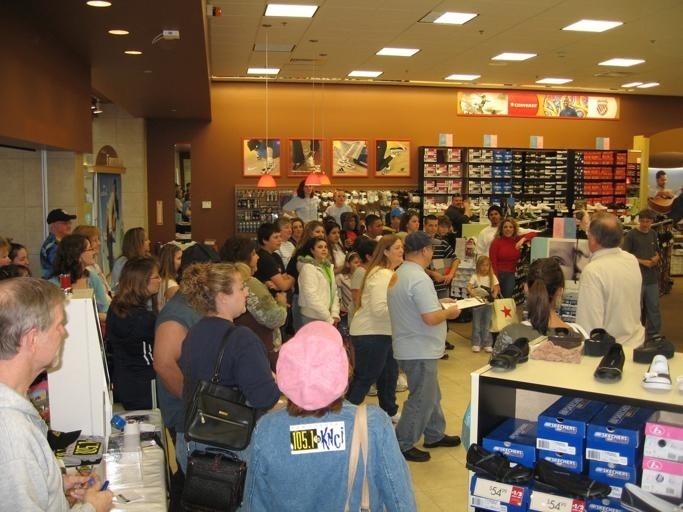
[472,345,480,352]
[620,483,683,512]
[642,355,671,391]
[484,347,492,352]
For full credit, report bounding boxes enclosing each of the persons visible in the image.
[153,244,227,472]
[624,210,660,340]
[576,218,646,349]
[0,181,586,419]
[647,171,674,214]
[386,232,461,462]
[177,261,288,511]
[0,276,114,512]
[240,320,416,511]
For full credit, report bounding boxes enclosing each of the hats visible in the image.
[47,430,81,449]
[47,209,77,224]
[403,232,441,252]
[276,322,349,411]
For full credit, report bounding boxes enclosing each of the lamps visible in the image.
[255,23,279,188]
[316,51,333,186]
[89,94,103,115]
[303,39,321,188]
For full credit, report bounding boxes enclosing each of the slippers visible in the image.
[548,326,582,349]
[633,335,673,363]
[584,328,615,356]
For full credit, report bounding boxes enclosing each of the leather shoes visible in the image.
[490,337,529,370]
[401,447,430,462]
[594,344,625,383]
[425,433,461,447]
[533,459,611,498]
[467,444,533,483]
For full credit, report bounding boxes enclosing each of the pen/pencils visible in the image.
[100,481,109,491]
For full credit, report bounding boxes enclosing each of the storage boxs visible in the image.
[471,395,682,512]
[520,296,576,327]
[552,217,578,239]
[424,149,626,223]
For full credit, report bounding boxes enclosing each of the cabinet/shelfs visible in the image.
[418,145,629,226]
[47,289,115,442]
[530,237,592,324]
[646,218,674,299]
[313,188,421,235]
[461,218,548,307]
[466,334,683,512]
[233,184,294,240]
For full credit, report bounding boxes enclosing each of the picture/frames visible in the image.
[329,137,369,179]
[371,136,413,179]
[240,136,282,178]
[285,136,327,176]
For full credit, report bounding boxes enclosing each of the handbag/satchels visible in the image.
[183,380,256,451]
[490,298,516,332]
[180,450,247,512]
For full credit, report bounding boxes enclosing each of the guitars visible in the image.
[648,187,683,214]
